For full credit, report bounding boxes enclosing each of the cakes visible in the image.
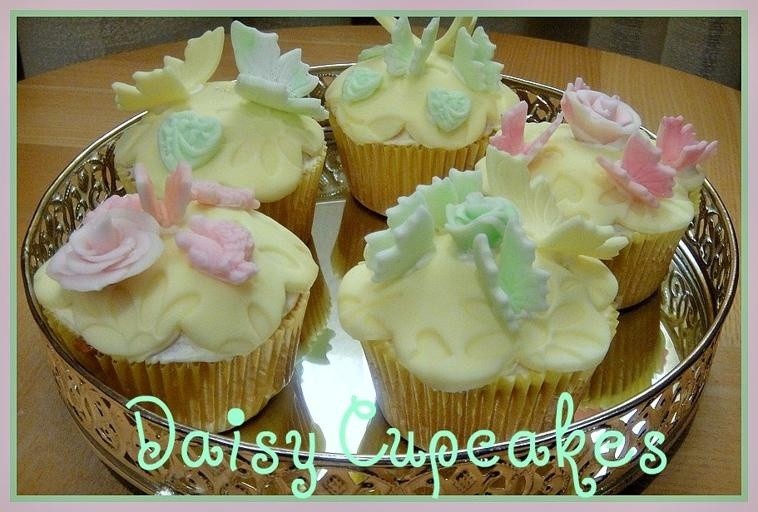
[112,21,329,246]
[324,15,521,218]
[298,235,335,366]
[571,285,669,423]
[475,75,719,310]
[33,157,320,434]
[226,367,327,471]
[335,144,628,456]
[329,193,389,281]
